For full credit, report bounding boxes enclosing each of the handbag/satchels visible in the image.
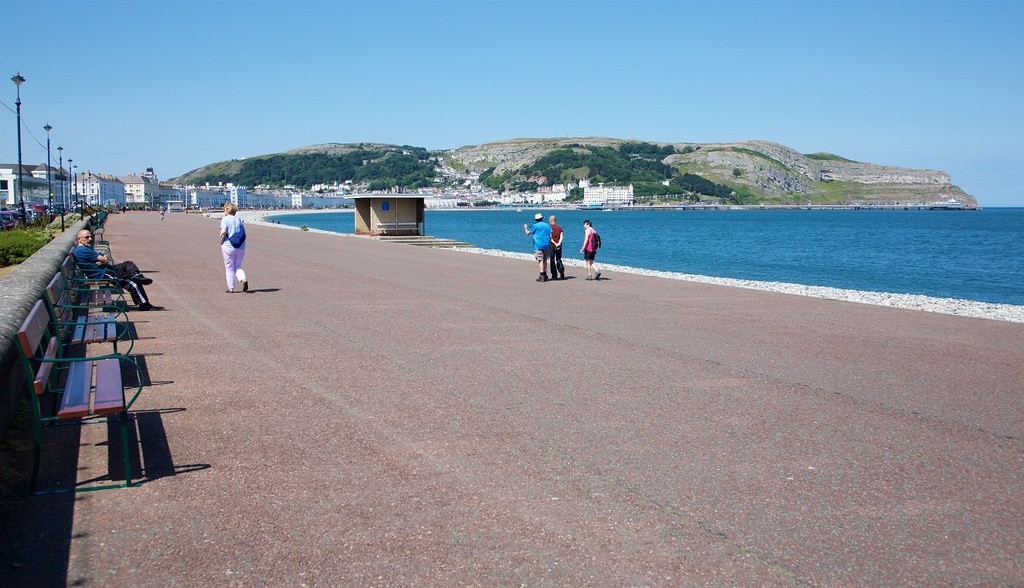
[224,218,246,249]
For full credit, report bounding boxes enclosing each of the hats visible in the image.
[534,214,543,220]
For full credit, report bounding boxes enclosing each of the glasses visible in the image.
[79,236,92,239]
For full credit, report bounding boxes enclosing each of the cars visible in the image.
[0,202,66,230]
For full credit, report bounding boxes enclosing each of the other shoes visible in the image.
[594,272,601,279]
[243,281,248,292]
[138,303,164,310]
[226,289,233,293]
[551,277,557,279]
[131,274,153,285]
[561,273,564,279]
[536,275,547,281]
[585,278,592,280]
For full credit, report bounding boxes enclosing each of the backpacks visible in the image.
[590,231,600,249]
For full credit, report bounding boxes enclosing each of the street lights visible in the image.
[10,73,28,230]
[67,159,73,211]
[74,166,78,208]
[56,146,65,232]
[43,122,54,222]
[82,172,85,203]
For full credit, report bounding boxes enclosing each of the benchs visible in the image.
[74,238,111,255]
[69,246,124,295]
[60,255,126,313]
[12,299,143,488]
[44,271,128,355]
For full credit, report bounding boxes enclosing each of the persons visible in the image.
[160,206,164,220]
[524,214,564,282]
[580,220,601,280]
[220,203,248,293]
[123,205,126,214]
[74,230,161,311]
